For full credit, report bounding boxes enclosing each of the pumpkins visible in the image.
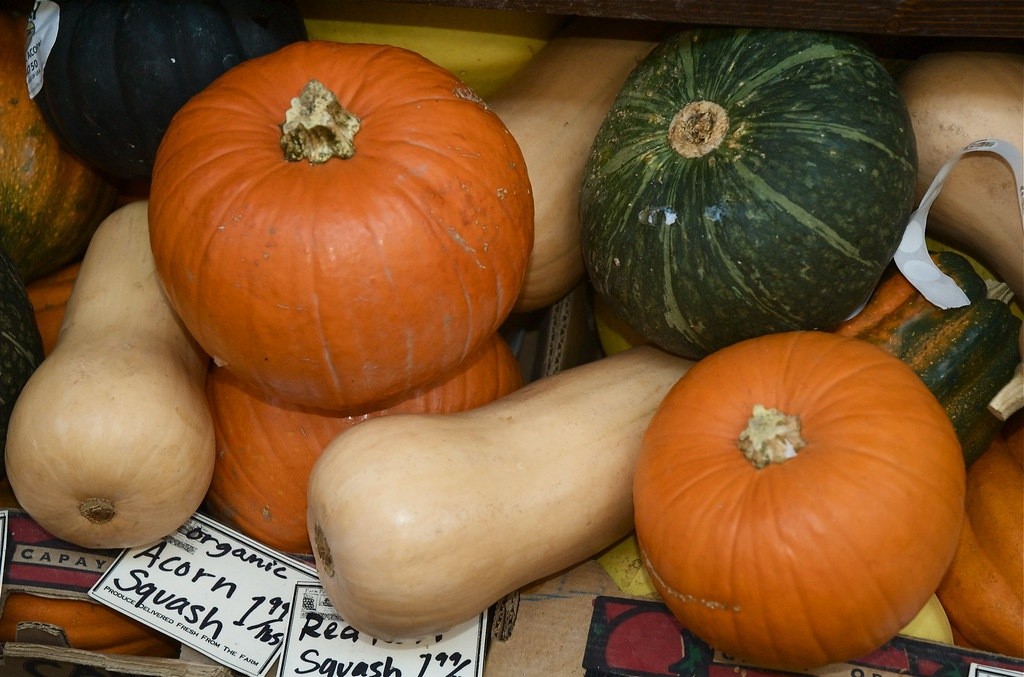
[0,0,1024,671]
